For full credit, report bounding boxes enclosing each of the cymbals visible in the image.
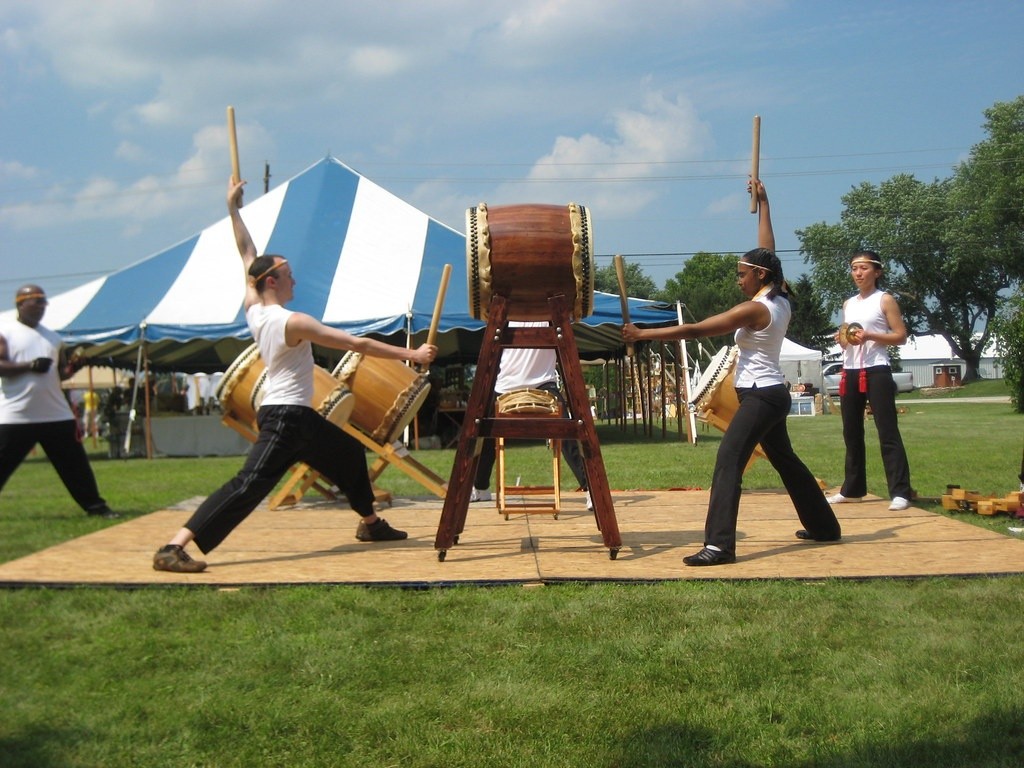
[839,322,864,349]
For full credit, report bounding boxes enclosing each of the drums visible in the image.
[331,349,431,444]
[463,200,596,322]
[688,345,741,424]
[213,341,356,434]
[496,388,559,413]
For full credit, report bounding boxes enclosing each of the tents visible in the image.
[711,337,824,398]
[0,152,700,446]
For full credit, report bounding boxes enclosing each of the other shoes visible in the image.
[88,505,120,519]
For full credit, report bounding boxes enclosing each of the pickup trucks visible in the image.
[821,363,914,398]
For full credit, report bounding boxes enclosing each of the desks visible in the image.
[440,409,467,448]
[102,413,145,458]
[143,416,254,458]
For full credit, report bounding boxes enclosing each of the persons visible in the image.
[83,377,220,439]
[153,176,439,573]
[0,285,121,519]
[825,250,911,511]
[469,321,594,510]
[623,173,841,566]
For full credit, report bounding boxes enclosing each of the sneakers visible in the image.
[356,517,408,541]
[586,491,594,511]
[888,497,910,509]
[469,487,492,502]
[826,493,861,503]
[152,544,206,572]
[682,548,735,566]
[795,528,813,540]
